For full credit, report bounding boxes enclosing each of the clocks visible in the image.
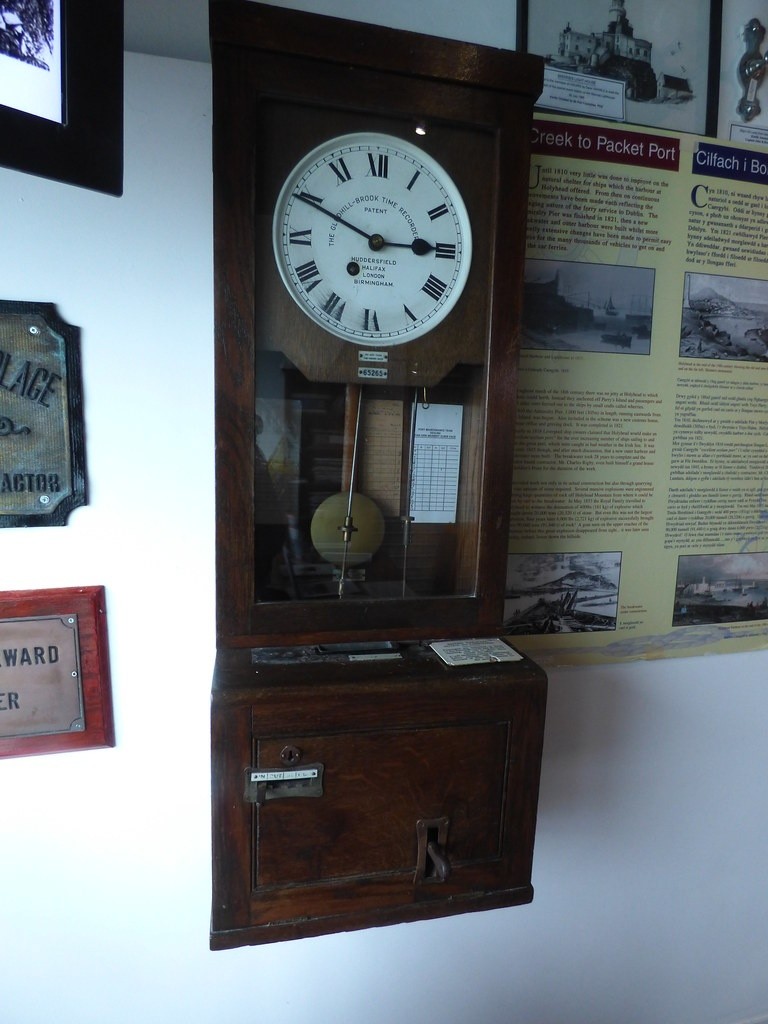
[210,0,545,649]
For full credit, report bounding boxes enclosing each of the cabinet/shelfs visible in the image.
[202,640,550,952]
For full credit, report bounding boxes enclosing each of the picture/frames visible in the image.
[0,0,125,196]
[517,0,722,137]
